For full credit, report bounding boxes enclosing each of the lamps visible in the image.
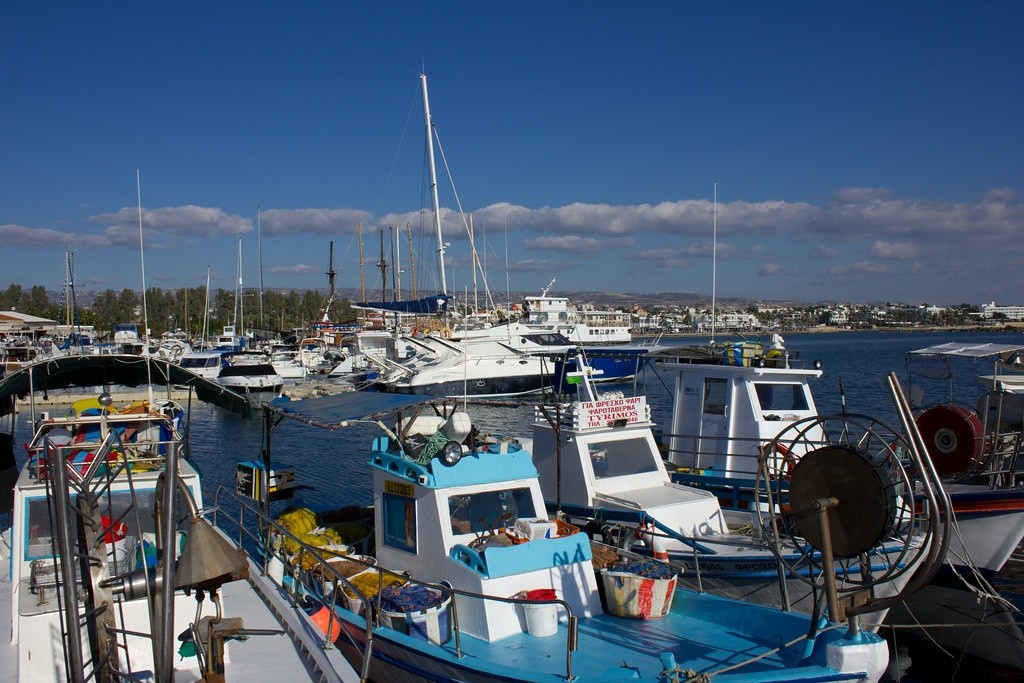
[437,440,462,467]
[813,359,822,370]
[611,419,629,428]
[755,358,765,369]
[98,392,113,420]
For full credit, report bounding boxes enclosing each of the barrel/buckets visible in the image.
[101,515,128,543]
[522,589,558,637]
[600,567,677,619]
[105,538,128,563]
[270,526,453,646]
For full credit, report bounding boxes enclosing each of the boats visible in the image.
[236,285,952,683]
[0,169,374,683]
[512,181,1024,633]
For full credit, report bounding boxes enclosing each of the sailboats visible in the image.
[0,72,763,398]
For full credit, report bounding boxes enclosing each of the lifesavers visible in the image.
[621,529,670,570]
[759,444,798,484]
[440,327,452,340]
